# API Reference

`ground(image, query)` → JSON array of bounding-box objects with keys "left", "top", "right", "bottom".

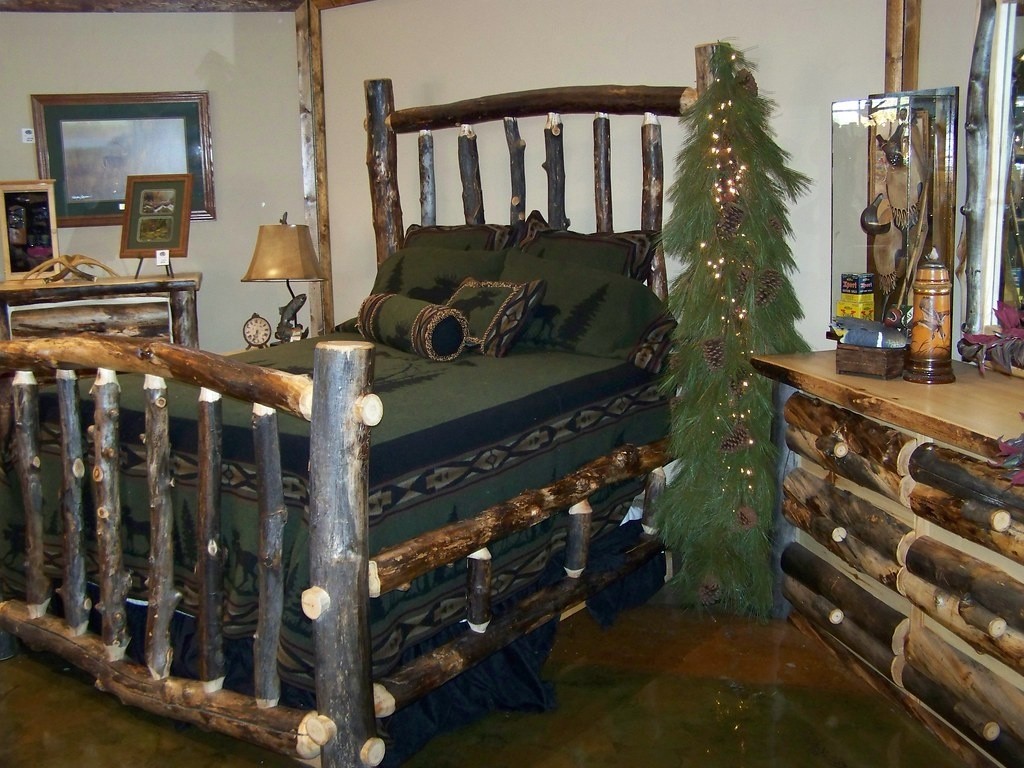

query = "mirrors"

[{"left": 955, "top": 0, "right": 1024, "bottom": 377}]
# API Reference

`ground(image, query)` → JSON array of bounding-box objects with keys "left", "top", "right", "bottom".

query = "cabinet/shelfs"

[
  {"left": 0, "top": 272, "right": 202, "bottom": 383},
  {"left": 750, "top": 350, "right": 1024, "bottom": 768}
]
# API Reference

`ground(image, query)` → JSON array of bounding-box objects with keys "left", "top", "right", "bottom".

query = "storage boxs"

[
  {"left": 837, "top": 273, "right": 874, "bottom": 321},
  {"left": 826, "top": 86, "right": 959, "bottom": 359},
  {"left": 836, "top": 344, "right": 904, "bottom": 380}
]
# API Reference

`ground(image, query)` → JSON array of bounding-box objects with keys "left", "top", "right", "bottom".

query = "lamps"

[{"left": 241, "top": 212, "right": 327, "bottom": 347}]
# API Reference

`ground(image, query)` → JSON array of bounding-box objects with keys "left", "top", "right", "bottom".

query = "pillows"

[{"left": 357, "top": 210, "right": 662, "bottom": 361}]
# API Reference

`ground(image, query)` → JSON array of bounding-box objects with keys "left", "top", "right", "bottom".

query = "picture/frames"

[
  {"left": 120, "top": 174, "right": 192, "bottom": 258},
  {"left": 31, "top": 90, "right": 216, "bottom": 228}
]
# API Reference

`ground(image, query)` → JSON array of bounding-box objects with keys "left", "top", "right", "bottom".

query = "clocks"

[{"left": 243, "top": 313, "right": 271, "bottom": 350}]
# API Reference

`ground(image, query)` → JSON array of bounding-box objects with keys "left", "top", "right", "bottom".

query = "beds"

[{"left": 0, "top": 42, "right": 776, "bottom": 768}]
